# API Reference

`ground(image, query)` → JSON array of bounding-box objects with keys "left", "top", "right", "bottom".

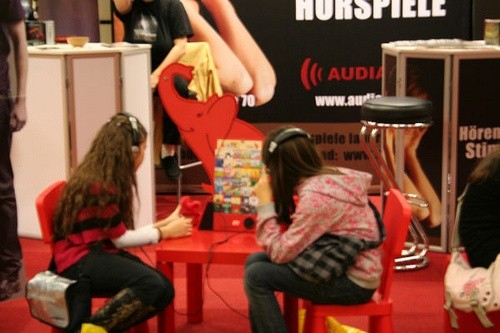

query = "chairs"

[
  {"left": 284, "top": 188, "right": 413, "bottom": 333},
  {"left": 34, "top": 179, "right": 72, "bottom": 275}
]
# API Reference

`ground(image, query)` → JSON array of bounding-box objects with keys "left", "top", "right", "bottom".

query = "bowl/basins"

[{"left": 66, "top": 36, "right": 89, "bottom": 47}]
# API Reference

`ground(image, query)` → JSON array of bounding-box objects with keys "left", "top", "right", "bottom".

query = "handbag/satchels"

[
  {"left": 443, "top": 251, "right": 500, "bottom": 330},
  {"left": 286, "top": 230, "right": 365, "bottom": 287},
  {"left": 25, "top": 270, "right": 81, "bottom": 331}
]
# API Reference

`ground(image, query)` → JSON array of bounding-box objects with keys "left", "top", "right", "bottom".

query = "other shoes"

[
  {"left": 159, "top": 152, "right": 180, "bottom": 178},
  {"left": 0, "top": 279, "right": 22, "bottom": 301}
]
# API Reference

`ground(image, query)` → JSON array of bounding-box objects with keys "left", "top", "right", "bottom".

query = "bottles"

[{"left": 28, "top": 0, "right": 39, "bottom": 20}]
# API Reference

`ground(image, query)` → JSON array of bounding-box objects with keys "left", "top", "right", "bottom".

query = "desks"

[
  {"left": 381, "top": 39, "right": 500, "bottom": 253},
  {"left": 12, "top": 40, "right": 156, "bottom": 240},
  {"left": 155, "top": 196, "right": 299, "bottom": 333}
]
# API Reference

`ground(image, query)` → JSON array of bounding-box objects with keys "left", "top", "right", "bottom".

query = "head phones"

[
  {"left": 265, "top": 128, "right": 311, "bottom": 173},
  {"left": 120, "top": 112, "right": 141, "bottom": 157}
]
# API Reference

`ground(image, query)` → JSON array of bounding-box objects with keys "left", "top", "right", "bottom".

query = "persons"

[
  {"left": 112, "top": 0, "right": 277, "bottom": 106},
  {"left": 0, "top": 0, "right": 28, "bottom": 301},
  {"left": 459, "top": 146, "right": 500, "bottom": 333},
  {"left": 124, "top": 0, "right": 188, "bottom": 181},
  {"left": 51, "top": 112, "right": 194, "bottom": 333},
  {"left": 242, "top": 123, "right": 383, "bottom": 333}
]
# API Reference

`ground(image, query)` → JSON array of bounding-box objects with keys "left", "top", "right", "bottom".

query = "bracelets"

[{"left": 157, "top": 227, "right": 163, "bottom": 243}]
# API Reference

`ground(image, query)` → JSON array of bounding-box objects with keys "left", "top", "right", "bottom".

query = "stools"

[{"left": 357, "top": 95, "right": 443, "bottom": 271}]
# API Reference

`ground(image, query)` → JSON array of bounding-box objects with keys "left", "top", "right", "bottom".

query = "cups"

[{"left": 484, "top": 19, "right": 500, "bottom": 45}]
